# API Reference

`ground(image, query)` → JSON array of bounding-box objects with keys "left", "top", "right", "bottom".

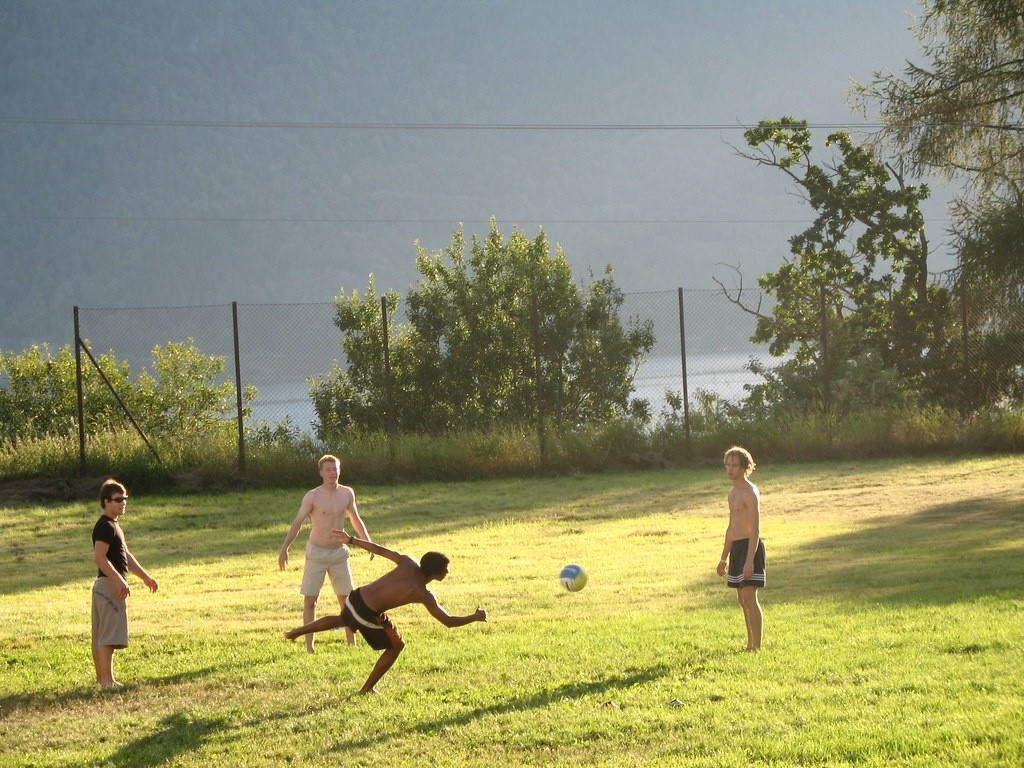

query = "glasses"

[{"left": 107, "top": 495, "right": 128, "bottom": 502}]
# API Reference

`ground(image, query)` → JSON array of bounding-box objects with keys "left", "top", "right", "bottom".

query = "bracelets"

[
  {"left": 720, "top": 560, "right": 728, "bottom": 563},
  {"left": 349, "top": 536, "right": 355, "bottom": 545}
]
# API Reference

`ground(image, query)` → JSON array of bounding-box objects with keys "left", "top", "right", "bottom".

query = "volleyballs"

[{"left": 559, "top": 564, "right": 588, "bottom": 592}]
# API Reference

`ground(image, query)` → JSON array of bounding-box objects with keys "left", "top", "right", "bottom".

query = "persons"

[
  {"left": 277, "top": 454, "right": 374, "bottom": 656},
  {"left": 282, "top": 529, "right": 489, "bottom": 697},
  {"left": 91, "top": 480, "right": 158, "bottom": 687},
  {"left": 717, "top": 448, "right": 766, "bottom": 650}
]
